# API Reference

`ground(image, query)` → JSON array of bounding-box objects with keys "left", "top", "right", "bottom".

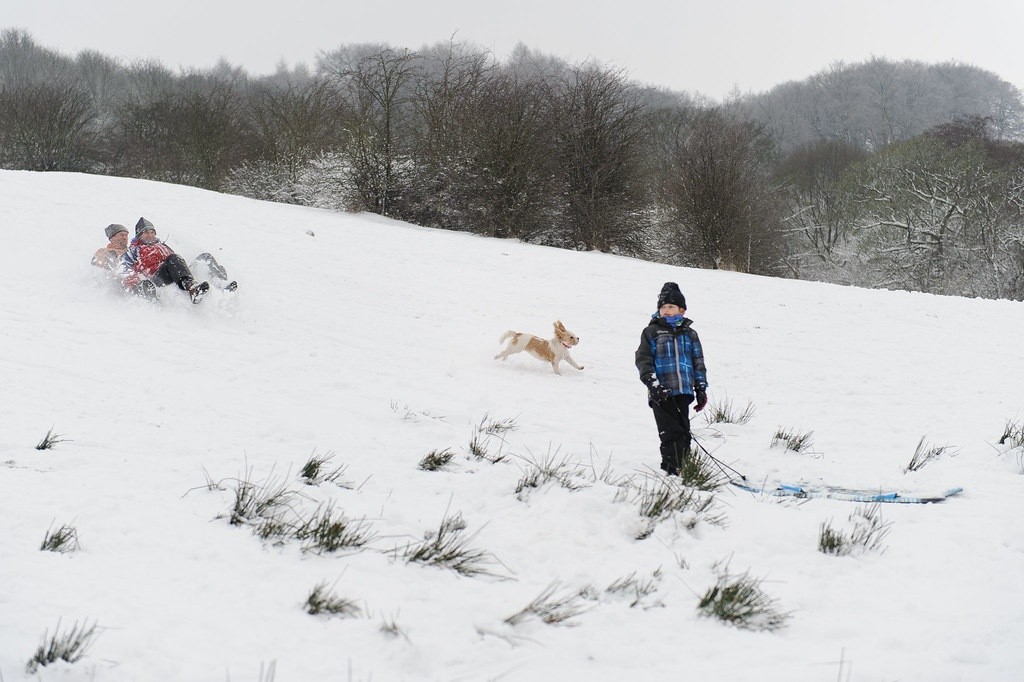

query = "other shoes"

[
  {"left": 142, "top": 280, "right": 162, "bottom": 303},
  {"left": 190, "top": 281, "right": 209, "bottom": 304},
  {"left": 225, "top": 281, "right": 237, "bottom": 292}
]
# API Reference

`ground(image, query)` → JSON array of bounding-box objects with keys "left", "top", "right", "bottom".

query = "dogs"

[{"left": 493, "top": 320, "right": 584, "bottom": 376}]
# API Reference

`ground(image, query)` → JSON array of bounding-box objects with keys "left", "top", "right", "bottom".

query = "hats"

[
  {"left": 136, "top": 217, "right": 156, "bottom": 237},
  {"left": 658, "top": 282, "right": 687, "bottom": 310},
  {"left": 105, "top": 224, "right": 129, "bottom": 240}
]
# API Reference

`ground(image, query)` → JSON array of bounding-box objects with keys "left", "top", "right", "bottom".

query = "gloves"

[
  {"left": 692, "top": 391, "right": 707, "bottom": 412},
  {"left": 650, "top": 385, "right": 672, "bottom": 406}
]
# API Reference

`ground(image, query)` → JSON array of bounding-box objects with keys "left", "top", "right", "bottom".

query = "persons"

[
  {"left": 91, "top": 217, "right": 238, "bottom": 307},
  {"left": 634, "top": 281, "right": 709, "bottom": 480}
]
